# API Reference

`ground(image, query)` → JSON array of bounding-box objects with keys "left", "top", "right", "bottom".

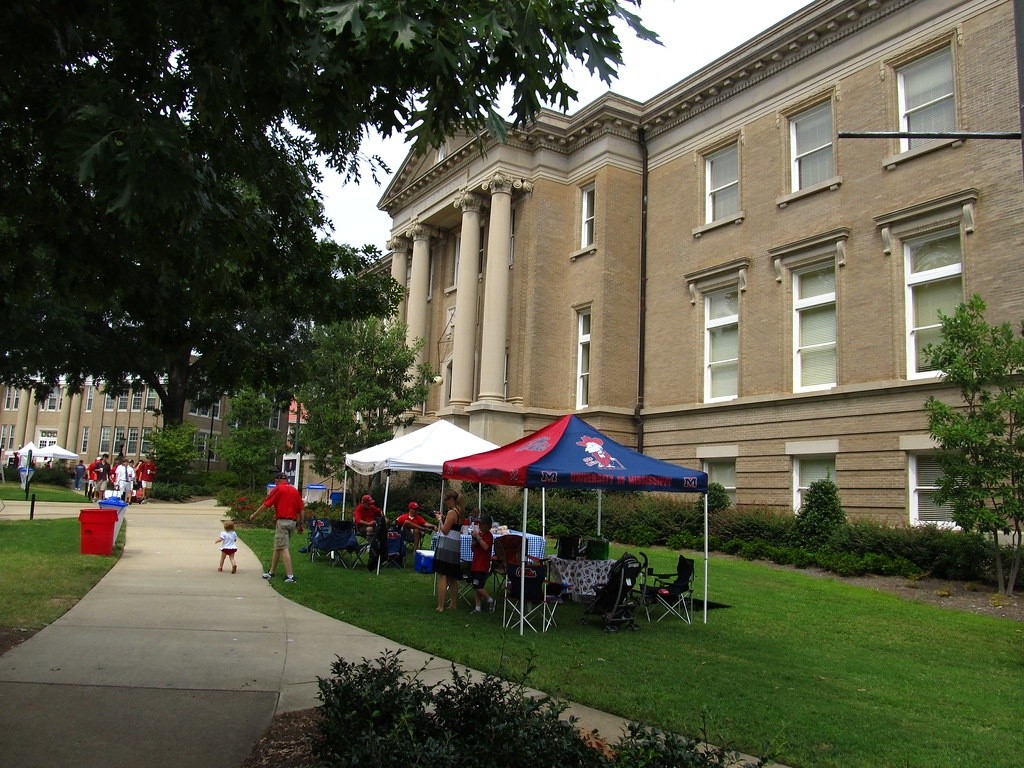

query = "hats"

[
  {"left": 408, "top": 502, "right": 419, "bottom": 509},
  {"left": 475, "top": 514, "right": 492, "bottom": 524},
  {"left": 361, "top": 495, "right": 375, "bottom": 504},
  {"left": 97, "top": 457, "right": 101, "bottom": 460},
  {"left": 275, "top": 473, "right": 288, "bottom": 479}
]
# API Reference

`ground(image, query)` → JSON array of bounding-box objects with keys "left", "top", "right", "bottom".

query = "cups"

[
  {"left": 112, "top": 475, "right": 114, "bottom": 478},
  {"left": 100, "top": 468, "right": 103, "bottom": 472},
  {"left": 435, "top": 511, "right": 440, "bottom": 519},
  {"left": 147, "top": 470, "right": 150, "bottom": 474}
]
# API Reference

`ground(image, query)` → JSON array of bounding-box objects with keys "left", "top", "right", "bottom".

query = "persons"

[
  {"left": 470, "top": 515, "right": 497, "bottom": 615},
  {"left": 353, "top": 495, "right": 389, "bottom": 554},
  {"left": 75, "top": 460, "right": 87, "bottom": 491},
  {"left": 249, "top": 472, "right": 305, "bottom": 584},
  {"left": 32, "top": 460, "right": 50, "bottom": 469},
  {"left": 392, "top": 501, "right": 437, "bottom": 555},
  {"left": 85, "top": 454, "right": 157, "bottom": 505},
  {"left": 216, "top": 521, "right": 237, "bottom": 574},
  {"left": 434, "top": 491, "right": 465, "bottom": 612},
  {"left": 12, "top": 452, "right": 19, "bottom": 467}
]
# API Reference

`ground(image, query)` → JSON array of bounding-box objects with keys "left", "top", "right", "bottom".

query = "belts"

[{"left": 276, "top": 518, "right": 296, "bottom": 521}]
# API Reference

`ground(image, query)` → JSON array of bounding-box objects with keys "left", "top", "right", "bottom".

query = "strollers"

[{"left": 576, "top": 552, "right": 649, "bottom": 633}]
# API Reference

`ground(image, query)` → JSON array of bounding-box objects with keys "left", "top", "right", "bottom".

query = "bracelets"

[
  {"left": 478, "top": 537, "right": 482, "bottom": 542},
  {"left": 438, "top": 518, "right": 441, "bottom": 521}
]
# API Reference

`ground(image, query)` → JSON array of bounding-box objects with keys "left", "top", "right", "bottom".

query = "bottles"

[{"left": 474, "top": 521, "right": 479, "bottom": 534}]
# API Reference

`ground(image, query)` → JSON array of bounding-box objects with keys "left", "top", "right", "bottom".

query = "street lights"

[{"left": 118, "top": 433, "right": 126, "bottom": 455}]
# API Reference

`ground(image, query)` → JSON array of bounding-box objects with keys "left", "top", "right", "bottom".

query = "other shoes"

[
  {"left": 434, "top": 607, "right": 444, "bottom": 614},
  {"left": 425, "top": 528, "right": 432, "bottom": 535},
  {"left": 219, "top": 568, "right": 222, "bottom": 571},
  {"left": 448, "top": 604, "right": 457, "bottom": 611},
  {"left": 367, "top": 546, "right": 370, "bottom": 554},
  {"left": 232, "top": 566, "right": 236, "bottom": 574},
  {"left": 413, "top": 550, "right": 416, "bottom": 556}
]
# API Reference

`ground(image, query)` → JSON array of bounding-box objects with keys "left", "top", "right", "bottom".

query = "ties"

[{"left": 125, "top": 466, "right": 128, "bottom": 482}]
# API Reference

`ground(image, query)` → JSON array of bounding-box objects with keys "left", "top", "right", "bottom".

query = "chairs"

[{"left": 307, "top": 516, "right": 694, "bottom": 632}]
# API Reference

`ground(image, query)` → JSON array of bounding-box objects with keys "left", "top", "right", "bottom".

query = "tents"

[
  {"left": 32, "top": 444, "right": 79, "bottom": 467},
  {"left": 434, "top": 414, "right": 708, "bottom": 623},
  {"left": 18, "top": 440, "right": 38, "bottom": 456},
  {"left": 341, "top": 418, "right": 502, "bottom": 534}
]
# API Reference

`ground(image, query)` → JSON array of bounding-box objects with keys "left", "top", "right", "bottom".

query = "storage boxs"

[{"left": 414, "top": 549, "right": 436, "bottom": 573}]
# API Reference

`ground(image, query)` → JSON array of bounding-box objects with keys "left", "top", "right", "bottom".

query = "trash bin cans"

[
  {"left": 330, "top": 491, "right": 343, "bottom": 507},
  {"left": 79, "top": 509, "right": 118, "bottom": 555}
]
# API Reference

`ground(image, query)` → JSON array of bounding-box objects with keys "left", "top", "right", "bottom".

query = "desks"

[
  {"left": 547, "top": 556, "right": 617, "bottom": 603},
  {"left": 431, "top": 530, "right": 545, "bottom": 562}
]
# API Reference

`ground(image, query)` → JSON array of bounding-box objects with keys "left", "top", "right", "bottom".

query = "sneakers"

[
  {"left": 487, "top": 598, "right": 496, "bottom": 614},
  {"left": 283, "top": 575, "right": 296, "bottom": 584},
  {"left": 262, "top": 570, "right": 274, "bottom": 580},
  {"left": 471, "top": 610, "right": 482, "bottom": 615}
]
